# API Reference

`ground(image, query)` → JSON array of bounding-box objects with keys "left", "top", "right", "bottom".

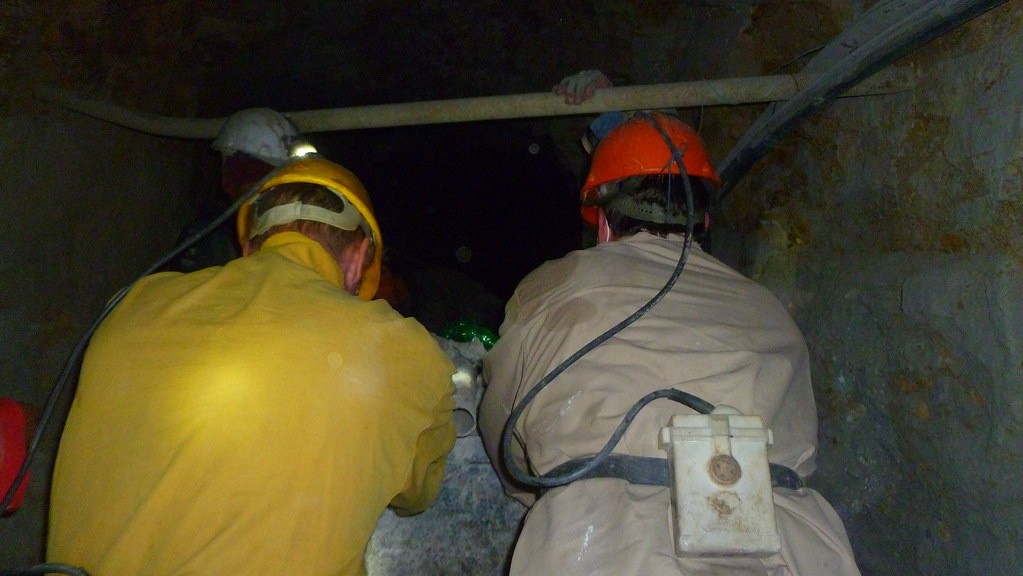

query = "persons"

[{"left": 44, "top": 70, "right": 860, "bottom": 576}]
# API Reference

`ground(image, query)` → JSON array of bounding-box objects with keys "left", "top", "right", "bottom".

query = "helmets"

[
  {"left": 580, "top": 113, "right": 720, "bottom": 223},
  {"left": 210, "top": 108, "right": 298, "bottom": 167},
  {"left": 237, "top": 155, "right": 382, "bottom": 300}
]
